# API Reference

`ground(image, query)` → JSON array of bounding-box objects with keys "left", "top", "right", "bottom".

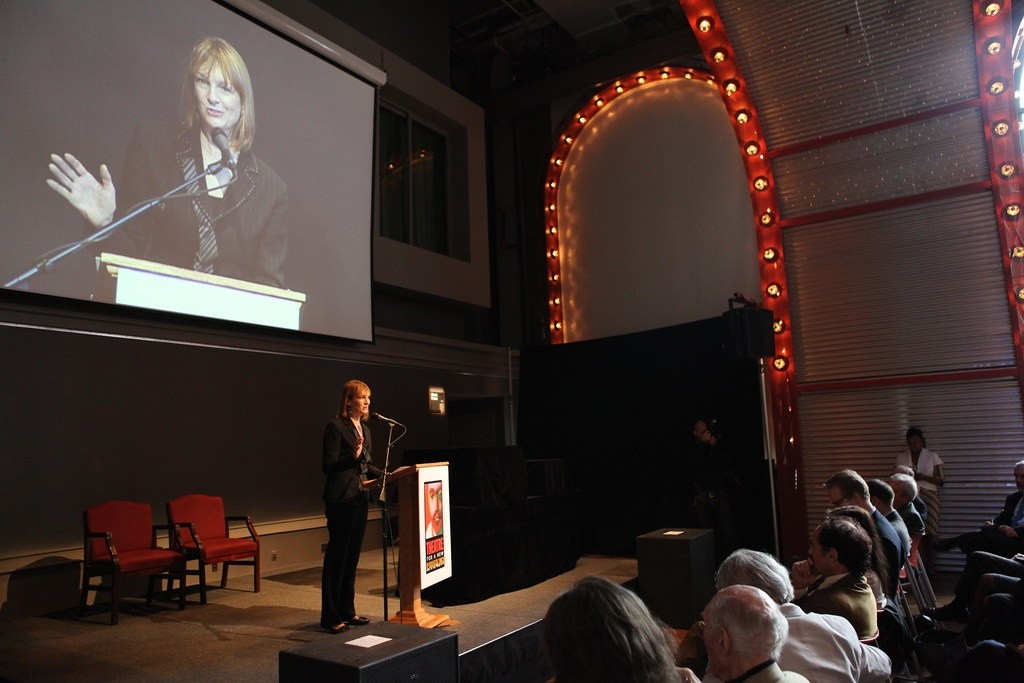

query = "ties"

[
  {"left": 1011, "top": 499, "right": 1024, "bottom": 528},
  {"left": 807, "top": 576, "right": 825, "bottom": 594}
]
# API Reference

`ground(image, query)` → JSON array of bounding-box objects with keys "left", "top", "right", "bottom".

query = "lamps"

[
  {"left": 549, "top": 204, "right": 556, "bottom": 214},
  {"left": 660, "top": 71, "right": 671, "bottom": 80},
  {"left": 997, "top": 161, "right": 1018, "bottom": 180},
  {"left": 684, "top": 71, "right": 694, "bottom": 80},
  {"left": 696, "top": 14, "right": 790, "bottom": 374},
  {"left": 550, "top": 180, "right": 557, "bottom": 190},
  {"left": 636, "top": 76, "right": 647, "bottom": 84},
  {"left": 552, "top": 272, "right": 560, "bottom": 282},
  {"left": 986, "top": 75, "right": 1010, "bottom": 97},
  {"left": 595, "top": 98, "right": 604, "bottom": 107},
  {"left": 982, "top": 36, "right": 1006, "bottom": 56},
  {"left": 979, "top": 0, "right": 1005, "bottom": 17},
  {"left": 991, "top": 119, "right": 1012, "bottom": 138},
  {"left": 554, "top": 321, "right": 562, "bottom": 331},
  {"left": 555, "top": 157, "right": 563, "bottom": 167},
  {"left": 564, "top": 135, "right": 573, "bottom": 144},
  {"left": 578, "top": 115, "right": 587, "bottom": 124},
  {"left": 1000, "top": 202, "right": 1024, "bottom": 222},
  {"left": 551, "top": 248, "right": 559, "bottom": 260},
  {"left": 550, "top": 226, "right": 557, "bottom": 236},
  {"left": 1015, "top": 285, "right": 1024, "bottom": 304},
  {"left": 553, "top": 295, "right": 560, "bottom": 307},
  {"left": 1010, "top": 243, "right": 1024, "bottom": 263},
  {"left": 615, "top": 85, "right": 625, "bottom": 94}
]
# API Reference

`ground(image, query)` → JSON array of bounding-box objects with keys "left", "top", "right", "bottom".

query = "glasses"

[
  {"left": 833, "top": 496, "right": 847, "bottom": 508},
  {"left": 699, "top": 621, "right": 718, "bottom": 632}
]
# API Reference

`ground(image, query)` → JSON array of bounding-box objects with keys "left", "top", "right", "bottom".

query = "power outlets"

[
  {"left": 271, "top": 550, "right": 278, "bottom": 562},
  {"left": 321, "top": 542, "right": 329, "bottom": 553},
  {"left": 211, "top": 563, "right": 218, "bottom": 572}
]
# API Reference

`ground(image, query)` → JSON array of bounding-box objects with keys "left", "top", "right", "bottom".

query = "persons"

[
  {"left": 320, "top": 379, "right": 411, "bottom": 634},
  {"left": 47, "top": 36, "right": 290, "bottom": 284},
  {"left": 687, "top": 420, "right": 740, "bottom": 552},
  {"left": 541, "top": 427, "right": 1024, "bottom": 683}
]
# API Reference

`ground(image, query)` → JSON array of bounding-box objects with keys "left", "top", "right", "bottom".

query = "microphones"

[
  {"left": 212, "top": 128, "right": 236, "bottom": 173},
  {"left": 372, "top": 413, "right": 397, "bottom": 426}
]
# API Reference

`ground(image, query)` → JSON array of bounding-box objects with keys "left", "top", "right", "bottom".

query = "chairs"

[
  {"left": 165, "top": 494, "right": 261, "bottom": 605},
  {"left": 78, "top": 499, "right": 187, "bottom": 627},
  {"left": 861, "top": 531, "right": 946, "bottom": 683}
]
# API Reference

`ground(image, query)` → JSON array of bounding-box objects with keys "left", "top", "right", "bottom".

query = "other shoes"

[
  {"left": 933, "top": 536, "right": 961, "bottom": 551},
  {"left": 321, "top": 623, "right": 350, "bottom": 634},
  {"left": 342, "top": 617, "right": 370, "bottom": 624},
  {"left": 922, "top": 604, "right": 970, "bottom": 624}
]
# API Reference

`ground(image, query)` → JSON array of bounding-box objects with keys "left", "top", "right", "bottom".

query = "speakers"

[
  {"left": 279, "top": 620, "right": 460, "bottom": 683},
  {"left": 638, "top": 526, "right": 717, "bottom": 629}
]
